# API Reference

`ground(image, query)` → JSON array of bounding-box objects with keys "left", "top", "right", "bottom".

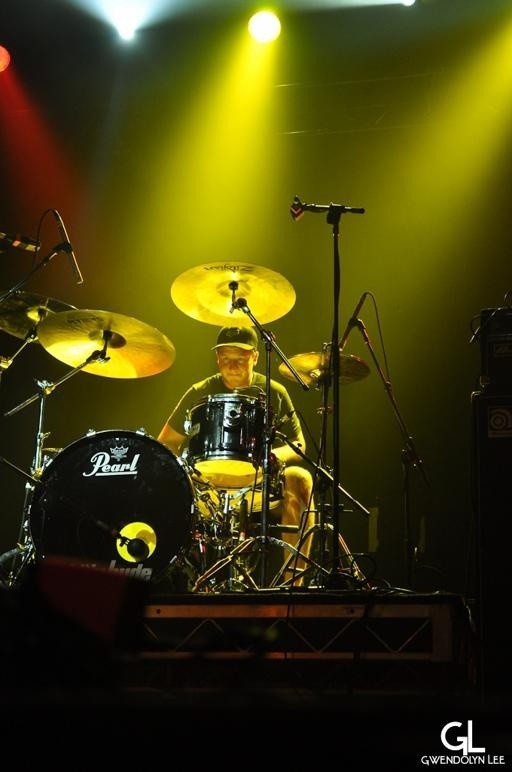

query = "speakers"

[{"left": 466, "top": 392, "right": 512, "bottom": 772}]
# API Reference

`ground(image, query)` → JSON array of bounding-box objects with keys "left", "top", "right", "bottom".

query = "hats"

[{"left": 211, "top": 326, "right": 258, "bottom": 351}]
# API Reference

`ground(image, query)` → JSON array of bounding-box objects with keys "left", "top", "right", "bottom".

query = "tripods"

[
  {"left": 190, "top": 309, "right": 328, "bottom": 591},
  {"left": 272, "top": 226, "right": 378, "bottom": 590}
]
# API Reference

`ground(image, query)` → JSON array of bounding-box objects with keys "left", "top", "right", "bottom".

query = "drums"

[
  {"left": 22, "top": 427, "right": 205, "bottom": 596},
  {"left": 187, "top": 393, "right": 268, "bottom": 490},
  {"left": 218, "top": 459, "right": 286, "bottom": 512},
  {"left": 30, "top": 445, "right": 62, "bottom": 493}
]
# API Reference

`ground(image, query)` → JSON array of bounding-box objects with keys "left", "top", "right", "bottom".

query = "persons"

[{"left": 158, "top": 323, "right": 315, "bottom": 588}]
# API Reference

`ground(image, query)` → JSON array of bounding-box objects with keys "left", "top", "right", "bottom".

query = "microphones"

[
  {"left": 236, "top": 296, "right": 248, "bottom": 313},
  {"left": 312, "top": 201, "right": 368, "bottom": 218},
  {"left": 56, "top": 210, "right": 84, "bottom": 283},
  {"left": 339, "top": 295, "right": 365, "bottom": 352}
]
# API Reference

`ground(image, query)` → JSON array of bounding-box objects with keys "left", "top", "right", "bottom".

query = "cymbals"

[
  {"left": 0, "top": 287, "right": 78, "bottom": 344},
  {"left": 170, "top": 261, "right": 296, "bottom": 327},
  {"left": 36, "top": 309, "right": 176, "bottom": 380},
  {"left": 278, "top": 351, "right": 369, "bottom": 387}
]
